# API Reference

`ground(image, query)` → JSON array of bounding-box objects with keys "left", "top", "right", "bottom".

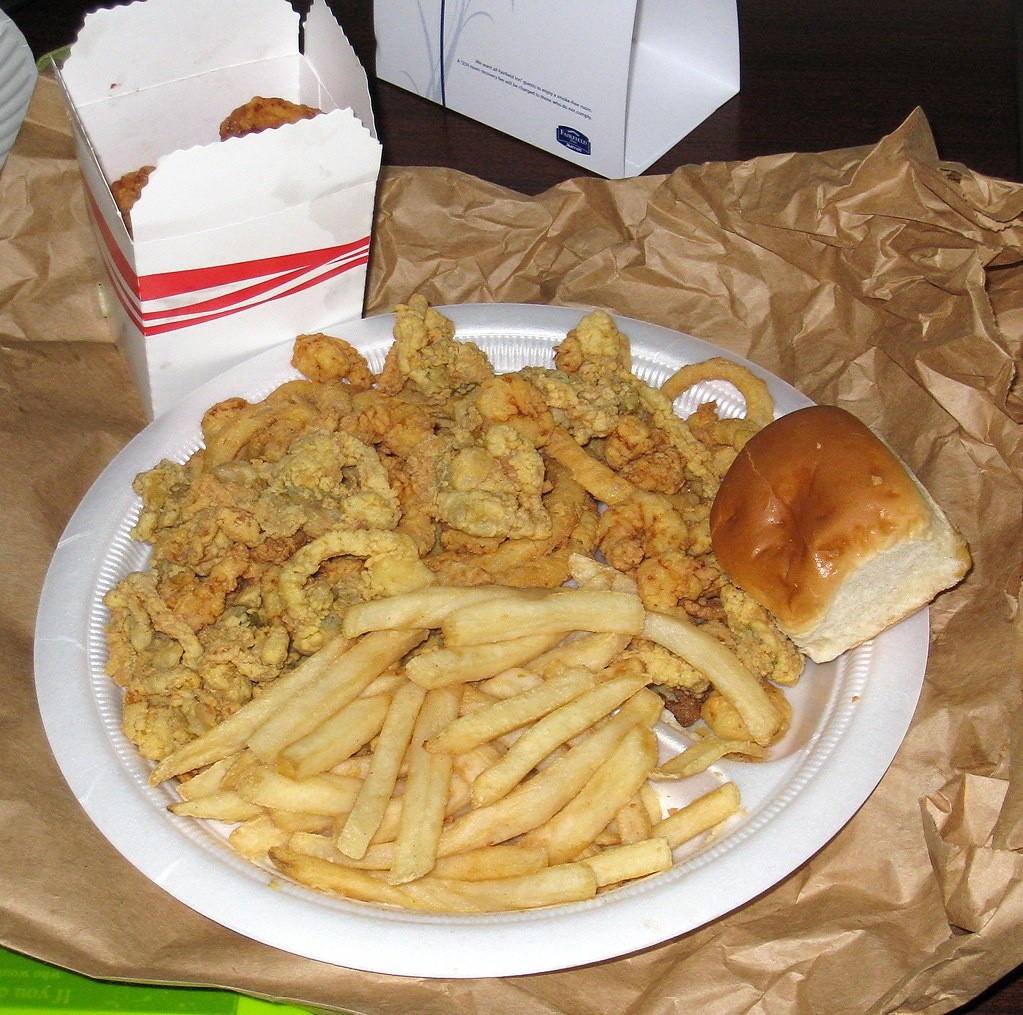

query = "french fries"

[{"left": 147, "top": 553, "right": 787, "bottom": 913}]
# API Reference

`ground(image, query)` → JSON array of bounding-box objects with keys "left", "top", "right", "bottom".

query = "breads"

[{"left": 712, "top": 403, "right": 973, "bottom": 664}]
